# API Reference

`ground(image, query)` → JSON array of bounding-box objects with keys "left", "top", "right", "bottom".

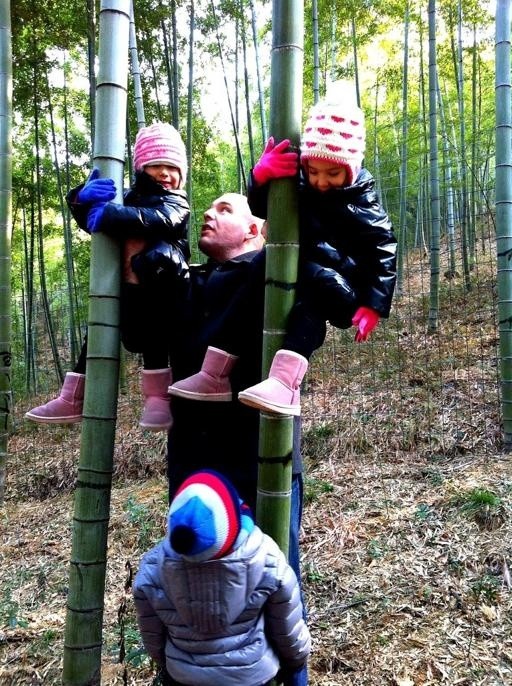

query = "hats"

[
  {"left": 299, "top": 80, "right": 365, "bottom": 186},
  {"left": 167, "top": 470, "right": 255, "bottom": 563},
  {"left": 134, "top": 122, "right": 188, "bottom": 188}
]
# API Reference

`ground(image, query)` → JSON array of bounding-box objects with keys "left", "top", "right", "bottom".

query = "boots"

[
  {"left": 168, "top": 346, "right": 238, "bottom": 402},
  {"left": 140, "top": 368, "right": 174, "bottom": 430},
  {"left": 238, "top": 349, "right": 309, "bottom": 416},
  {"left": 26, "top": 372, "right": 85, "bottom": 423}
]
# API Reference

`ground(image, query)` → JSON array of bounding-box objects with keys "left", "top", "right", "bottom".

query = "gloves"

[
  {"left": 253, "top": 135, "right": 298, "bottom": 185},
  {"left": 78, "top": 169, "right": 116, "bottom": 231},
  {"left": 352, "top": 307, "right": 379, "bottom": 342}
]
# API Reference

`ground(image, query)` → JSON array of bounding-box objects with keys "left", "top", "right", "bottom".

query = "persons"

[
  {"left": 166, "top": 96, "right": 398, "bottom": 418},
  {"left": 168, "top": 193, "right": 305, "bottom": 686},
  {"left": 131, "top": 470, "right": 312, "bottom": 686},
  {"left": 26, "top": 120, "right": 190, "bottom": 432}
]
